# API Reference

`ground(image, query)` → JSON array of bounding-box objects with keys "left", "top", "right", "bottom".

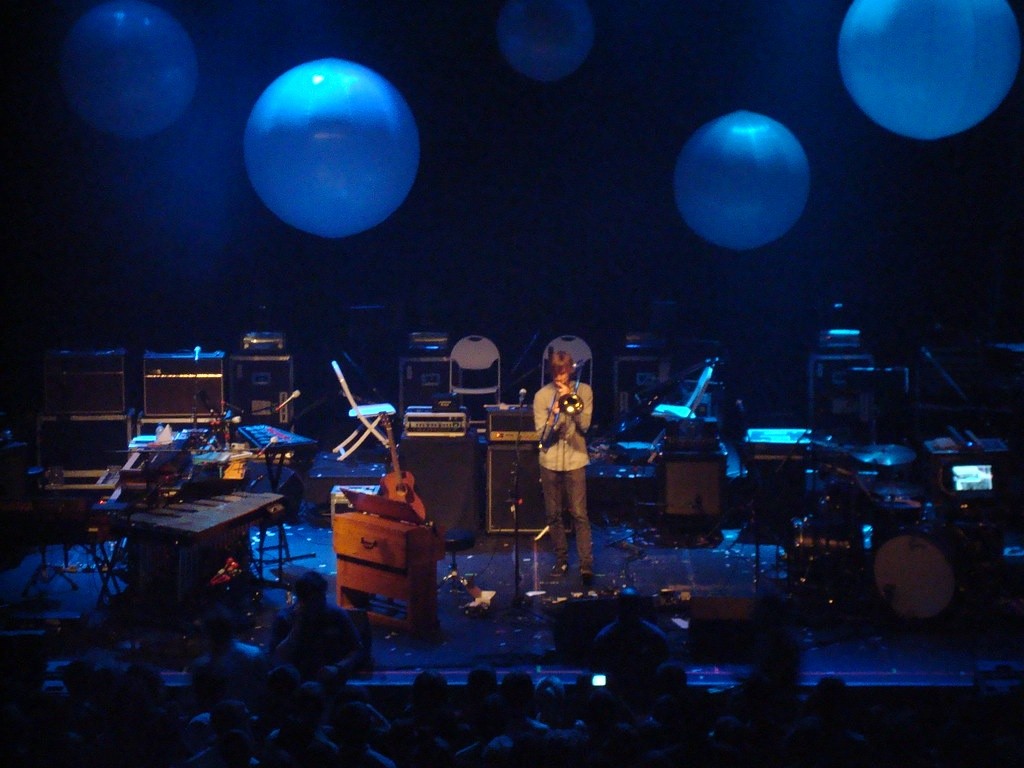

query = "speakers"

[
  {"left": 144, "top": 350, "right": 227, "bottom": 416},
  {"left": 653, "top": 451, "right": 724, "bottom": 518},
  {"left": 42, "top": 349, "right": 131, "bottom": 414},
  {"left": 555, "top": 595, "right": 656, "bottom": 656},
  {"left": 686, "top": 595, "right": 759, "bottom": 651},
  {"left": 487, "top": 444, "right": 574, "bottom": 534}
]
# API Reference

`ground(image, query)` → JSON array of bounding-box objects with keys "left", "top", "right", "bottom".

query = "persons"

[
  {"left": 532, "top": 350, "right": 594, "bottom": 586},
  {"left": 0, "top": 570, "right": 1024, "bottom": 768}
]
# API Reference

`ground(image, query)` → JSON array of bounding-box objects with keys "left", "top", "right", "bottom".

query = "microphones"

[
  {"left": 194, "top": 347, "right": 202, "bottom": 361},
  {"left": 210, "top": 416, "right": 241, "bottom": 425},
  {"left": 519, "top": 388, "right": 527, "bottom": 402},
  {"left": 275, "top": 390, "right": 301, "bottom": 411}
]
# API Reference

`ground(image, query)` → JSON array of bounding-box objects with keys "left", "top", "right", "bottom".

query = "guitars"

[{"left": 378, "top": 412, "right": 427, "bottom": 519}]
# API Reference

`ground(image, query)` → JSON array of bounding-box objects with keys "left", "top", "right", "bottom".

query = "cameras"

[{"left": 590, "top": 675, "right": 607, "bottom": 686}]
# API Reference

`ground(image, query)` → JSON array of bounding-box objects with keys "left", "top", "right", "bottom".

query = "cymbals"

[
  {"left": 872, "top": 481, "right": 923, "bottom": 497},
  {"left": 849, "top": 441, "right": 916, "bottom": 467},
  {"left": 878, "top": 498, "right": 922, "bottom": 509}
]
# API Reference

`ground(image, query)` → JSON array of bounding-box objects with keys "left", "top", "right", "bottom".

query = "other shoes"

[
  {"left": 580, "top": 566, "right": 592, "bottom": 578},
  {"left": 551, "top": 559, "right": 568, "bottom": 577}
]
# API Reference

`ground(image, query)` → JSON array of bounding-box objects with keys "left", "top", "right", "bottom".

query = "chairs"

[
  {"left": 330, "top": 360, "right": 396, "bottom": 461},
  {"left": 651, "top": 364, "right": 714, "bottom": 449},
  {"left": 450, "top": 334, "right": 502, "bottom": 407},
  {"left": 540, "top": 336, "right": 594, "bottom": 387}
]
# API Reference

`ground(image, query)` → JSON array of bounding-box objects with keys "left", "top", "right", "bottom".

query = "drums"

[{"left": 872, "top": 520, "right": 971, "bottom": 622}]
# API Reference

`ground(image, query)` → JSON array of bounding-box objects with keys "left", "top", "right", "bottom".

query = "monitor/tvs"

[{"left": 951, "top": 464, "right": 994, "bottom": 492}]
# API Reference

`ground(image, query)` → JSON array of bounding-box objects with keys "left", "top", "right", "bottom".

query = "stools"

[{"left": 91, "top": 503, "right": 131, "bottom": 611}]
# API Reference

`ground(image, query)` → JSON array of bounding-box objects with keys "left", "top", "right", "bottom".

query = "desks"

[
  {"left": 33, "top": 408, "right": 134, "bottom": 463},
  {"left": 136, "top": 409, "right": 234, "bottom": 446}
]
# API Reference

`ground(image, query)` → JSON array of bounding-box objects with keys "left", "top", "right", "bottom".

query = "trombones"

[{"left": 537, "top": 357, "right": 584, "bottom": 446}]
